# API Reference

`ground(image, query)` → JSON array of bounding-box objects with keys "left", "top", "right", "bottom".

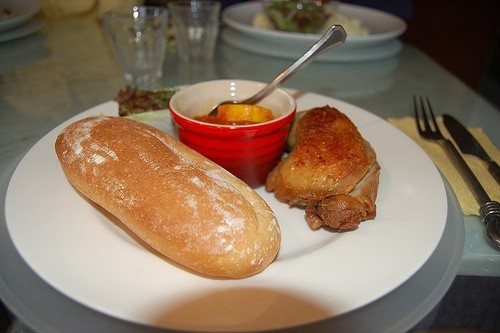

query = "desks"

[{"left": 0, "top": 13, "right": 500, "bottom": 275}]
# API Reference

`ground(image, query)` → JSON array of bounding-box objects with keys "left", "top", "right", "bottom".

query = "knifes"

[{"left": 440, "top": 112, "right": 500, "bottom": 185}]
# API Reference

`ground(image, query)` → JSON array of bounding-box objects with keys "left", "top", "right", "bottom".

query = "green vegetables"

[
  {"left": 264, "top": 0, "right": 326, "bottom": 33},
  {"left": 126, "top": 85, "right": 181, "bottom": 114}
]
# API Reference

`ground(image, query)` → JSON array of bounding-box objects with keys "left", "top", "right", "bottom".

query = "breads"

[{"left": 55, "top": 115, "right": 281, "bottom": 278}]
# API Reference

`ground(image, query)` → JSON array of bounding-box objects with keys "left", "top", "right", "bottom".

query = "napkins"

[{"left": 389, "top": 116, "right": 500, "bottom": 216}]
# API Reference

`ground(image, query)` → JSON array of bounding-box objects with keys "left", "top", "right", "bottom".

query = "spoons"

[{"left": 207, "top": 24, "right": 347, "bottom": 118}]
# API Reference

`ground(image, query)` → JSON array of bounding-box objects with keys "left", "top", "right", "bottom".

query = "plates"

[
  {"left": 222, "top": 0, "right": 406, "bottom": 50},
  {"left": 0, "top": 6, "right": 40, "bottom": 30},
  {"left": 5, "top": 83, "right": 447, "bottom": 333}
]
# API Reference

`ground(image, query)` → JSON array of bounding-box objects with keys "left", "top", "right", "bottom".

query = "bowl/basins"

[{"left": 168, "top": 78, "right": 296, "bottom": 191}]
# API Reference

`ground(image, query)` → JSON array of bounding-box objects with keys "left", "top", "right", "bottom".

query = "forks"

[{"left": 411, "top": 94, "right": 500, "bottom": 246}]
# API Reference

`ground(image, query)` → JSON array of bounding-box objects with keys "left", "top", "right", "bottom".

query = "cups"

[
  {"left": 102, "top": 6, "right": 168, "bottom": 84},
  {"left": 168, "top": 0, "right": 221, "bottom": 62}
]
